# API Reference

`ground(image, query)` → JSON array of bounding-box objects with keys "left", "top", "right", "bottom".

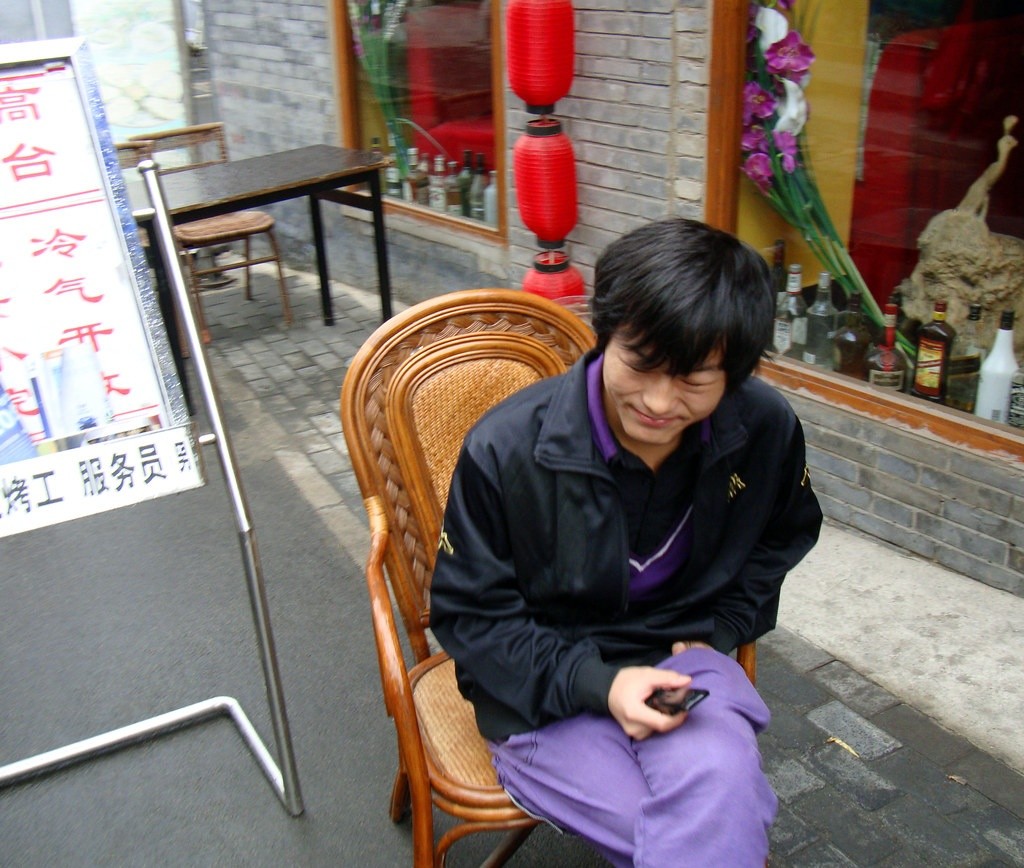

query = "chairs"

[
  {"left": 340, "top": 288, "right": 756, "bottom": 868},
  {"left": 131, "top": 114, "right": 292, "bottom": 344},
  {"left": 115, "top": 140, "right": 156, "bottom": 289}
]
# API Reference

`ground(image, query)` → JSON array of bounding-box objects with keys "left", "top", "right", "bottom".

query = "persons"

[{"left": 427, "top": 217, "right": 823, "bottom": 868}]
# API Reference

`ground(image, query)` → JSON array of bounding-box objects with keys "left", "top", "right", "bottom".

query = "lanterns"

[
  {"left": 505, "top": 0, "right": 575, "bottom": 114},
  {"left": 514, "top": 119, "right": 578, "bottom": 248},
  {"left": 522, "top": 250, "right": 586, "bottom": 318}
]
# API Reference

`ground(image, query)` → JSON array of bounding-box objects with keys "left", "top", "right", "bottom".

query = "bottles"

[
  {"left": 484, "top": 170, "right": 498, "bottom": 226},
  {"left": 416, "top": 152, "right": 431, "bottom": 206},
  {"left": 370, "top": 137, "right": 387, "bottom": 194},
  {"left": 766, "top": 238, "right": 787, "bottom": 299},
  {"left": 866, "top": 303, "right": 907, "bottom": 393},
  {"left": 429, "top": 156, "right": 447, "bottom": 213},
  {"left": 445, "top": 161, "right": 463, "bottom": 216},
  {"left": 836, "top": 291, "right": 868, "bottom": 334},
  {"left": 889, "top": 291, "right": 907, "bottom": 329},
  {"left": 802, "top": 272, "right": 837, "bottom": 371},
  {"left": 469, "top": 153, "right": 489, "bottom": 220},
  {"left": 833, "top": 302, "right": 868, "bottom": 381},
  {"left": 386, "top": 133, "right": 402, "bottom": 195},
  {"left": 403, "top": 148, "right": 419, "bottom": 203},
  {"left": 459, "top": 151, "right": 473, "bottom": 217},
  {"left": 944, "top": 304, "right": 981, "bottom": 414},
  {"left": 910, "top": 300, "right": 957, "bottom": 405},
  {"left": 776, "top": 265, "right": 808, "bottom": 360},
  {"left": 973, "top": 310, "right": 1019, "bottom": 423}
]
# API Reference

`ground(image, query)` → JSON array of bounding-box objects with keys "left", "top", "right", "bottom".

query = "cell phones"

[{"left": 645, "top": 688, "right": 709, "bottom": 715}]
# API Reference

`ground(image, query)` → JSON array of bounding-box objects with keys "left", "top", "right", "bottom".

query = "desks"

[{"left": 118, "top": 143, "right": 393, "bottom": 416}]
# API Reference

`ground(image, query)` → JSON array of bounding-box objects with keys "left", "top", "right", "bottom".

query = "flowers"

[
  {"left": 741, "top": 0, "right": 915, "bottom": 353},
  {"left": 348, "top": 0, "right": 410, "bottom": 179}
]
